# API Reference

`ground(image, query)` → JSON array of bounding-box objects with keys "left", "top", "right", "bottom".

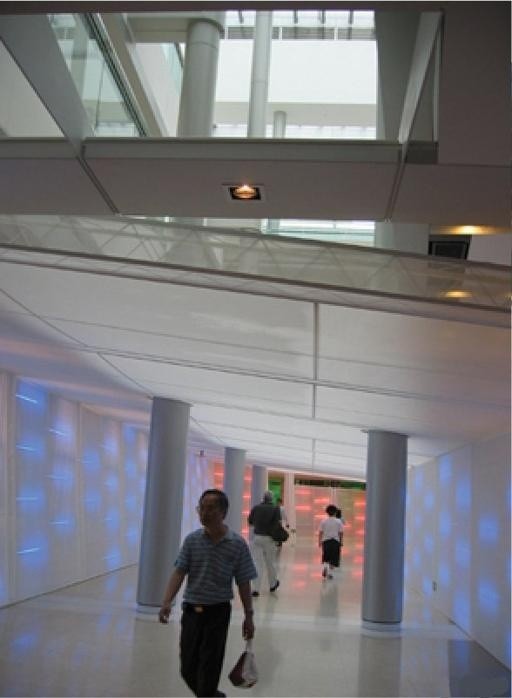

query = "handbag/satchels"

[{"left": 271, "top": 519, "right": 289, "bottom": 542}]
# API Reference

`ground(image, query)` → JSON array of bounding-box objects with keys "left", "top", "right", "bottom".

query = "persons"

[
  {"left": 254, "top": 596, "right": 286, "bottom": 697},
  {"left": 317, "top": 504, "right": 344, "bottom": 580},
  {"left": 313, "top": 580, "right": 341, "bottom": 653},
  {"left": 158, "top": 488, "right": 258, "bottom": 697},
  {"left": 335, "top": 508, "right": 344, "bottom": 525},
  {"left": 272, "top": 497, "right": 290, "bottom": 547},
  {"left": 246, "top": 490, "right": 282, "bottom": 596}
]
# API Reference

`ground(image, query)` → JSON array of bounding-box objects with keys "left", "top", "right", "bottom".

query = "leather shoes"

[
  {"left": 270, "top": 580, "right": 279, "bottom": 591},
  {"left": 252, "top": 591, "right": 258, "bottom": 596}
]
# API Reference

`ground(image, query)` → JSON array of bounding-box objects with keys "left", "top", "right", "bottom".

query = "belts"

[{"left": 185, "top": 603, "right": 214, "bottom": 613}]
